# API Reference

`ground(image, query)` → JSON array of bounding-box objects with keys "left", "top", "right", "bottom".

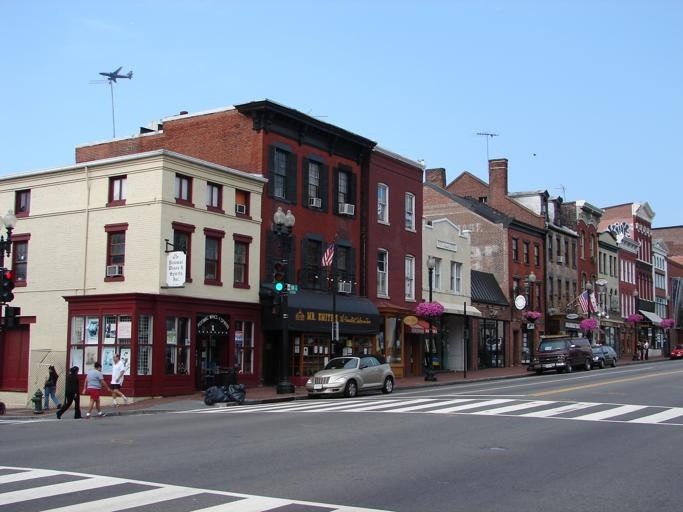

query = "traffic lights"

[
  {"left": 0, "top": 267, "right": 15, "bottom": 302},
  {"left": 7, "top": 307, "right": 21, "bottom": 328},
  {"left": 271, "top": 262, "right": 287, "bottom": 294}
]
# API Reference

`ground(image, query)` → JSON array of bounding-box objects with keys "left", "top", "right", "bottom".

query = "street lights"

[
  {"left": 585, "top": 280, "right": 592, "bottom": 337},
  {"left": 426, "top": 257, "right": 436, "bottom": 381},
  {"left": 271, "top": 207, "right": 295, "bottom": 394},
  {"left": 528, "top": 271, "right": 536, "bottom": 371},
  {"left": 632, "top": 288, "right": 639, "bottom": 361},
  {"left": 1, "top": 209, "right": 17, "bottom": 268}
]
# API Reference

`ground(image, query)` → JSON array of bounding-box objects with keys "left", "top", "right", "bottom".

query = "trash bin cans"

[{"left": 218, "top": 367, "right": 237, "bottom": 391}]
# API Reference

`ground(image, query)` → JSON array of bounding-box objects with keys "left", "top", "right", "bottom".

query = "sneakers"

[
  {"left": 96, "top": 413, "right": 105, "bottom": 417},
  {"left": 86, "top": 413, "right": 91, "bottom": 417}
]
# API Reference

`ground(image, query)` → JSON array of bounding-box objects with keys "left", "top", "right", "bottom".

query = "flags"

[
  {"left": 588, "top": 292, "right": 598, "bottom": 314},
  {"left": 318, "top": 241, "right": 335, "bottom": 267},
  {"left": 576, "top": 291, "right": 591, "bottom": 313}
]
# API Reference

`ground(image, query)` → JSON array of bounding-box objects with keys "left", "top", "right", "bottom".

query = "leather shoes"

[
  {"left": 74, "top": 417, "right": 82, "bottom": 419},
  {"left": 56, "top": 412, "right": 60, "bottom": 419}
]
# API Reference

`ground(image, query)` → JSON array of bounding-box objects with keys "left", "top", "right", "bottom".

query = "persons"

[
  {"left": 642, "top": 339, "right": 649, "bottom": 360},
  {"left": 41, "top": 365, "right": 62, "bottom": 411},
  {"left": 635, "top": 338, "right": 643, "bottom": 360},
  {"left": 108, "top": 353, "right": 129, "bottom": 410},
  {"left": 80, "top": 361, "right": 112, "bottom": 418},
  {"left": 55, "top": 365, "right": 84, "bottom": 419}
]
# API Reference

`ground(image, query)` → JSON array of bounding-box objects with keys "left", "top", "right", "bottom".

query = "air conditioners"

[
  {"left": 308, "top": 198, "right": 321, "bottom": 208},
  {"left": 338, "top": 283, "right": 351, "bottom": 293},
  {"left": 107, "top": 265, "right": 122, "bottom": 276},
  {"left": 235, "top": 204, "right": 246, "bottom": 214},
  {"left": 557, "top": 256, "right": 565, "bottom": 263},
  {"left": 339, "top": 204, "right": 355, "bottom": 216}
]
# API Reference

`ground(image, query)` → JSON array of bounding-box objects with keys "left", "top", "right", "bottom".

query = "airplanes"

[{"left": 99, "top": 66, "right": 134, "bottom": 83}]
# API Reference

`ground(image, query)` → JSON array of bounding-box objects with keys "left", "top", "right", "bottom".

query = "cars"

[
  {"left": 304, "top": 355, "right": 396, "bottom": 398},
  {"left": 486, "top": 337, "right": 502, "bottom": 352},
  {"left": 670, "top": 344, "right": 683, "bottom": 359},
  {"left": 533, "top": 337, "right": 617, "bottom": 375}
]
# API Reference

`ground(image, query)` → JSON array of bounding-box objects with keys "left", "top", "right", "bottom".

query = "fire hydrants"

[{"left": 31, "top": 389, "right": 44, "bottom": 414}]
